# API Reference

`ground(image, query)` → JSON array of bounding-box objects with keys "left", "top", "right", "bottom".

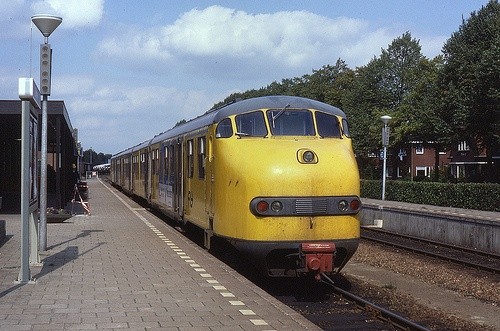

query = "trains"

[{"left": 109, "top": 95, "right": 363, "bottom": 283}]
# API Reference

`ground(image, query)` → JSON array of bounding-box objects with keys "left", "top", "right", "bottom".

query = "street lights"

[
  {"left": 30, "top": 14, "right": 63, "bottom": 251},
  {"left": 380, "top": 115, "right": 392, "bottom": 200}
]
